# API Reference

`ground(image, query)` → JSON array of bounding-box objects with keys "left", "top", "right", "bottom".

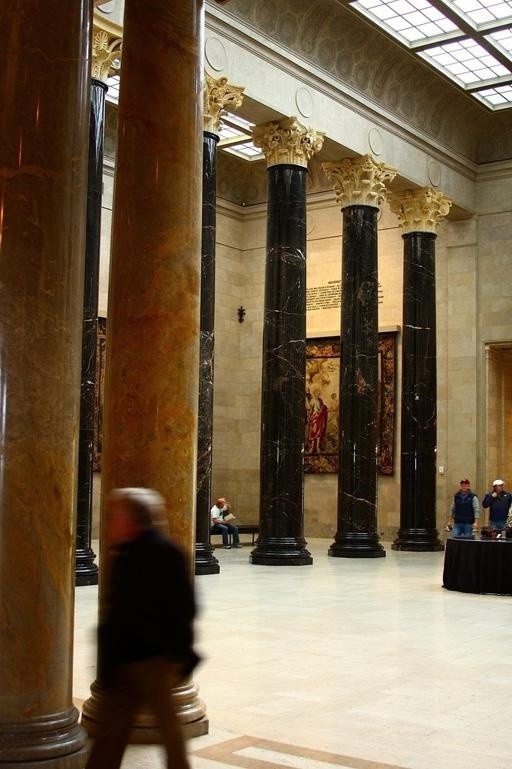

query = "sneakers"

[{"left": 222, "top": 544, "right": 243, "bottom": 549}]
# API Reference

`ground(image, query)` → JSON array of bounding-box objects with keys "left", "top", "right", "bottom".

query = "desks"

[{"left": 446, "top": 538, "right": 512, "bottom": 595}]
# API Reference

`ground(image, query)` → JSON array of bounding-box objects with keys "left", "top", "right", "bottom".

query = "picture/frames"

[{"left": 303, "top": 333, "right": 397, "bottom": 475}]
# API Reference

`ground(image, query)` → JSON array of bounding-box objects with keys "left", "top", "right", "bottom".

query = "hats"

[
  {"left": 492, "top": 480, "right": 505, "bottom": 487},
  {"left": 460, "top": 479, "right": 469, "bottom": 484}
]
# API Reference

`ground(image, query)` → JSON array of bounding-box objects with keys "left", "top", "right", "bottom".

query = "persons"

[
  {"left": 447, "top": 479, "right": 480, "bottom": 537},
  {"left": 210, "top": 498, "right": 243, "bottom": 549},
  {"left": 482, "top": 479, "right": 512, "bottom": 529},
  {"left": 86, "top": 488, "right": 200, "bottom": 768}
]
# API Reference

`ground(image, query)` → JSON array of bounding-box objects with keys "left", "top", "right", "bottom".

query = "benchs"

[{"left": 210, "top": 525, "right": 259, "bottom": 546}]
